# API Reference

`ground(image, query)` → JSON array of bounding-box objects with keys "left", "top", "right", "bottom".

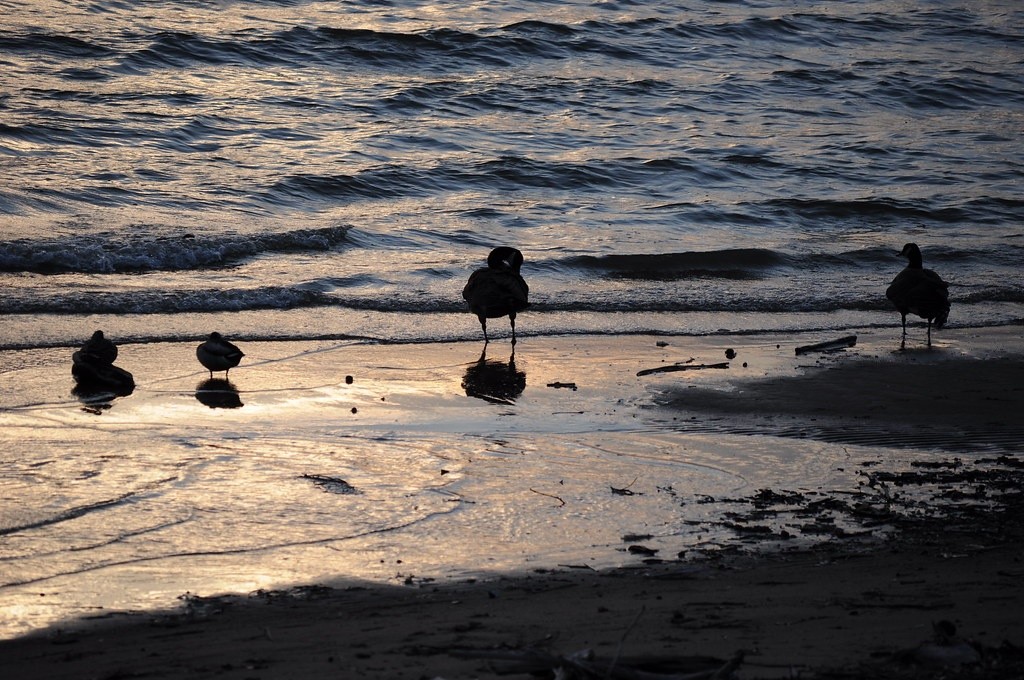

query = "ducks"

[
  {"left": 460, "top": 245, "right": 530, "bottom": 346},
  {"left": 884, "top": 241, "right": 953, "bottom": 349}
]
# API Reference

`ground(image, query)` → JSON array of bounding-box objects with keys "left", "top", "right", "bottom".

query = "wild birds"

[
  {"left": 72, "top": 329, "right": 119, "bottom": 368},
  {"left": 196, "top": 332, "right": 246, "bottom": 379}
]
